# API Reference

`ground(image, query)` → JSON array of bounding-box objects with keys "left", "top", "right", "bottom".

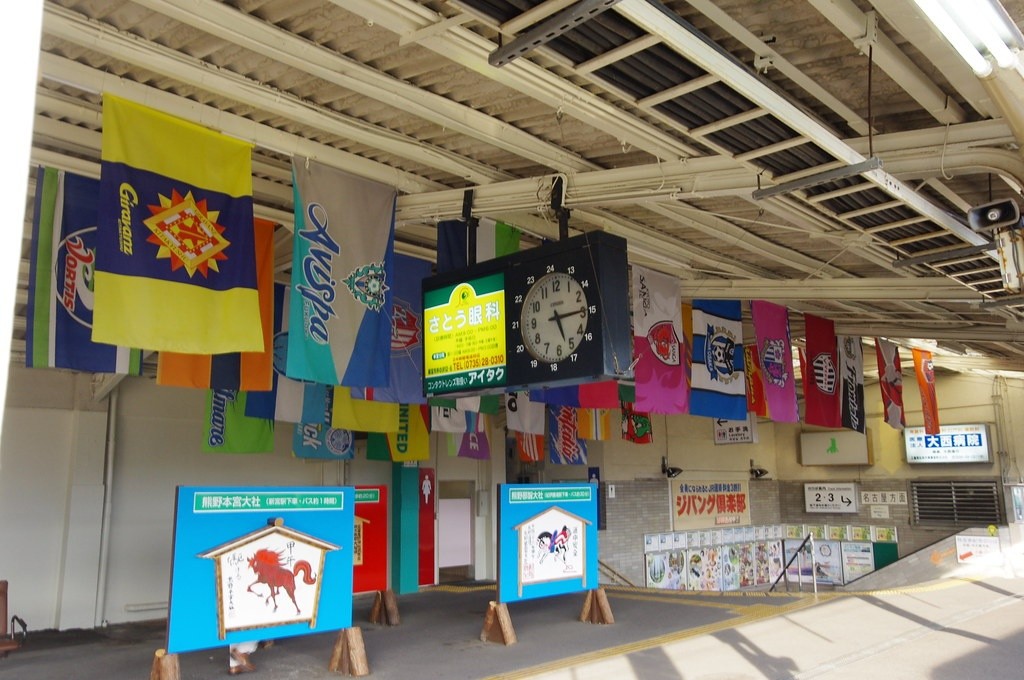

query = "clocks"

[{"left": 520, "top": 272, "right": 588, "bottom": 364}]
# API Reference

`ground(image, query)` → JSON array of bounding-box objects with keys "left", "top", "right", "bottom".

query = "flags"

[
  {"left": 201, "top": 250, "right": 652, "bottom": 466},
  {"left": 744, "top": 344, "right": 769, "bottom": 417},
  {"left": 875, "top": 337, "right": 906, "bottom": 429},
  {"left": 437, "top": 215, "right": 520, "bottom": 274},
  {"left": 153, "top": 216, "right": 273, "bottom": 390},
  {"left": 90, "top": 94, "right": 266, "bottom": 355},
  {"left": 284, "top": 156, "right": 395, "bottom": 386},
  {"left": 689, "top": 298, "right": 747, "bottom": 421},
  {"left": 804, "top": 312, "right": 842, "bottom": 428},
  {"left": 750, "top": 299, "right": 799, "bottom": 424},
  {"left": 913, "top": 349, "right": 940, "bottom": 434},
  {"left": 26, "top": 166, "right": 142, "bottom": 379},
  {"left": 633, "top": 264, "right": 688, "bottom": 415},
  {"left": 835, "top": 334, "right": 865, "bottom": 433},
  {"left": 797, "top": 348, "right": 805, "bottom": 398}
]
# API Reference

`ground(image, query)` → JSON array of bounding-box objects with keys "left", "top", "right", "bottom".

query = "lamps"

[
  {"left": 749, "top": 459, "right": 768, "bottom": 478},
  {"left": 661, "top": 455, "right": 684, "bottom": 478}
]
